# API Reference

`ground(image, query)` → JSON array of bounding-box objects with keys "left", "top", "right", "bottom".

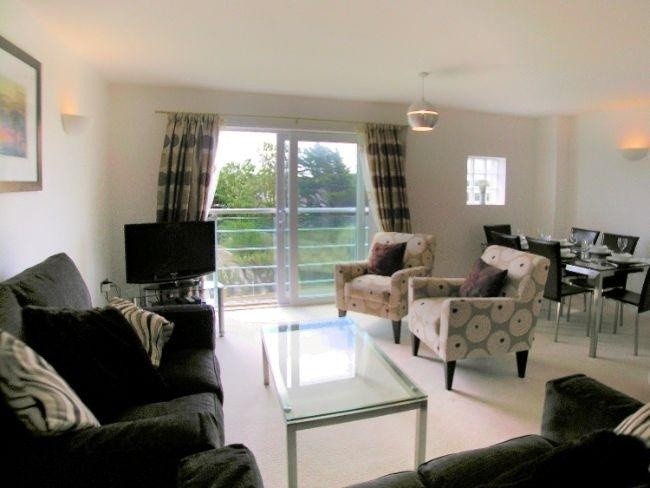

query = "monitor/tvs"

[{"left": 124, "top": 220, "right": 215, "bottom": 289}]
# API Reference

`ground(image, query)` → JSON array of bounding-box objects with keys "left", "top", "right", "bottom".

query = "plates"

[
  {"left": 608, "top": 256, "right": 641, "bottom": 264},
  {"left": 560, "top": 252, "right": 575, "bottom": 258},
  {"left": 561, "top": 243, "right": 573, "bottom": 247},
  {"left": 584, "top": 260, "right": 618, "bottom": 271},
  {"left": 588, "top": 248, "right": 612, "bottom": 256}
]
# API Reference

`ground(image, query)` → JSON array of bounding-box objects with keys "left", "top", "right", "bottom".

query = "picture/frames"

[{"left": 0, "top": 37, "right": 42, "bottom": 193}]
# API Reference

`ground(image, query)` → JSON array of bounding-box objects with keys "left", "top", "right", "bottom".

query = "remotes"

[
  {"left": 191, "top": 295, "right": 201, "bottom": 303},
  {"left": 184, "top": 295, "right": 195, "bottom": 304}
]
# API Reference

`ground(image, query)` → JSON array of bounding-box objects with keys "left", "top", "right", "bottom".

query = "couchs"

[
  {"left": 0, "top": 252, "right": 263, "bottom": 487},
  {"left": 344, "top": 373, "right": 650, "bottom": 488}
]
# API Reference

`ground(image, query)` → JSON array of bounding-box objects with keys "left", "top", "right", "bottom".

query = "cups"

[
  {"left": 560, "top": 248, "right": 570, "bottom": 255},
  {"left": 560, "top": 239, "right": 567, "bottom": 245},
  {"left": 581, "top": 251, "right": 589, "bottom": 260},
  {"left": 582, "top": 240, "right": 588, "bottom": 249}
]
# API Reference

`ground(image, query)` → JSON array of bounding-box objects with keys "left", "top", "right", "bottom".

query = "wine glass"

[{"left": 617, "top": 237, "right": 628, "bottom": 253}]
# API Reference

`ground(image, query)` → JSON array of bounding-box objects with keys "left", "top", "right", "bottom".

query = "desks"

[
  {"left": 127, "top": 280, "right": 226, "bottom": 337},
  {"left": 258, "top": 316, "right": 428, "bottom": 488}
]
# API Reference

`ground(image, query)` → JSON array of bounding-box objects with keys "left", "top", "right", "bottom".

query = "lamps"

[{"left": 406, "top": 71, "right": 439, "bottom": 131}]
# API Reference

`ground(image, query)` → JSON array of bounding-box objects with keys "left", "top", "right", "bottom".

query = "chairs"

[
  {"left": 335, "top": 231, "right": 435, "bottom": 343},
  {"left": 483, "top": 224, "right": 650, "bottom": 358},
  {"left": 407, "top": 245, "right": 551, "bottom": 391}
]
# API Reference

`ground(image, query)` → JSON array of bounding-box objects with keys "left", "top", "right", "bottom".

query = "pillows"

[
  {"left": 1, "top": 330, "right": 100, "bottom": 434},
  {"left": 110, "top": 297, "right": 175, "bottom": 369},
  {"left": 487, "top": 430, "right": 649, "bottom": 488},
  {"left": 25, "top": 306, "right": 165, "bottom": 419},
  {"left": 367, "top": 242, "right": 407, "bottom": 275},
  {"left": 457, "top": 258, "right": 507, "bottom": 297}
]
